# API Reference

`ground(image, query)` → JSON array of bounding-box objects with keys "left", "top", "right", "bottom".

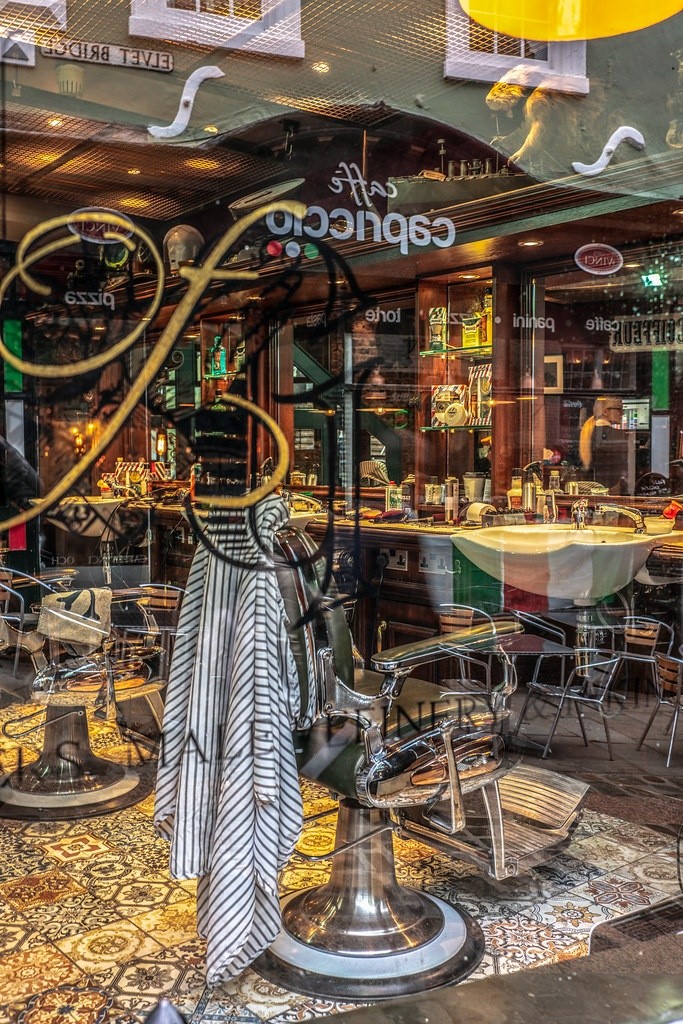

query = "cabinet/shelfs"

[
  {"left": 200, "top": 316, "right": 267, "bottom": 503},
  {"left": 416, "top": 263, "right": 522, "bottom": 523}
]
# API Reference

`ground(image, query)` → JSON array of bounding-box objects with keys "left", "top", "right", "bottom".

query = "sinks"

[{"left": 450, "top": 522, "right": 655, "bottom": 600}]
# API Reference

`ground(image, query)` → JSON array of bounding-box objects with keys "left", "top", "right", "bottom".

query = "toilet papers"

[{"left": 467, "top": 502, "right": 497, "bottom": 521}]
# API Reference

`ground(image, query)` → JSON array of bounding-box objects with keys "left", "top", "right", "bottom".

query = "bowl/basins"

[{"left": 644, "top": 517, "right": 675, "bottom": 535}]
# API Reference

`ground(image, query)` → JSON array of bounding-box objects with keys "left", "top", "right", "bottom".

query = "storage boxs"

[
  {"left": 461, "top": 318, "right": 482, "bottom": 353},
  {"left": 430, "top": 364, "right": 493, "bottom": 426}
]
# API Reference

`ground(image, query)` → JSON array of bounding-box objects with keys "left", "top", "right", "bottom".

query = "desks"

[{"left": 449, "top": 633, "right": 576, "bottom": 752}]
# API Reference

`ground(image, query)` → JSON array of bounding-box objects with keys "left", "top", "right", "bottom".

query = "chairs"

[
  {"left": 424, "top": 588, "right": 683, "bottom": 768},
  {"left": 0, "top": 562, "right": 197, "bottom": 819},
  {"left": 200, "top": 525, "right": 525, "bottom": 1000}
]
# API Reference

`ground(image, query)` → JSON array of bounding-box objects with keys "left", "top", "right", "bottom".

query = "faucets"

[{"left": 595, "top": 501, "right": 646, "bottom": 534}]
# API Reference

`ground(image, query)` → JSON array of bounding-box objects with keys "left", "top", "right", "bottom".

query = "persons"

[
  {"left": 579, "top": 396, "right": 627, "bottom": 490},
  {"left": 162, "top": 225, "right": 205, "bottom": 271}
]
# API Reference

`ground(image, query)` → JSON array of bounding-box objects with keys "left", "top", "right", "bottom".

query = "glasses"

[{"left": 606, "top": 406, "right": 624, "bottom": 415}]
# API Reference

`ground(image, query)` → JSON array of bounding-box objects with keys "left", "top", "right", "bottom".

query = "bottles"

[
  {"left": 292, "top": 466, "right": 301, "bottom": 474},
  {"left": 307, "top": 469, "right": 318, "bottom": 486}
]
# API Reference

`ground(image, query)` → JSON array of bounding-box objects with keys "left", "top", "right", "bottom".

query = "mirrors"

[{"left": 527, "top": 247, "right": 683, "bottom": 498}]
